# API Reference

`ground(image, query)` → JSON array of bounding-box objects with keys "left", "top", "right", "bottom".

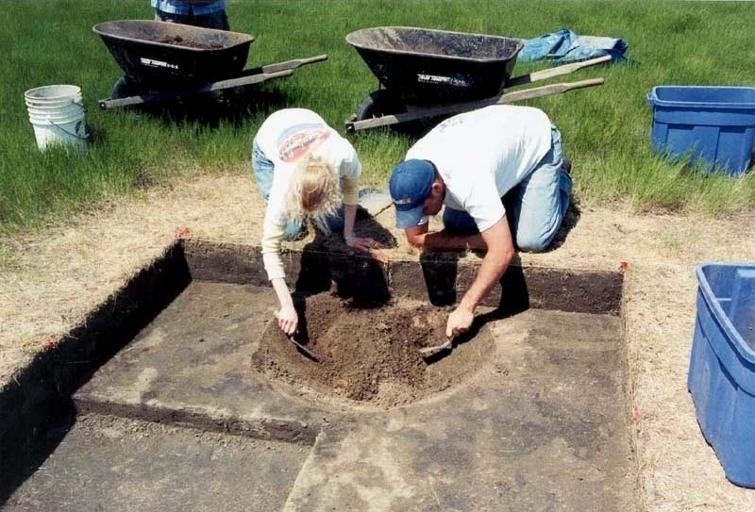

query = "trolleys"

[
  {"left": 90, "top": 14, "right": 332, "bottom": 113},
  {"left": 339, "top": 21, "right": 615, "bottom": 135}
]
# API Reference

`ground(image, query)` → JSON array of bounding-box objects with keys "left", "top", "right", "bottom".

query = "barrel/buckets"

[{"left": 25, "top": 84, "right": 88, "bottom": 154}]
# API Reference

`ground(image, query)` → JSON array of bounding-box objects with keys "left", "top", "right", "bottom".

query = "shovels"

[{"left": 273, "top": 309, "right": 321, "bottom": 361}]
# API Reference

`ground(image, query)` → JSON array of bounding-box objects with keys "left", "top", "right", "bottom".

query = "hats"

[{"left": 389, "top": 159, "right": 436, "bottom": 229}]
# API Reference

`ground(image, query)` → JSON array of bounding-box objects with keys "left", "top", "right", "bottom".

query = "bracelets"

[{"left": 345, "top": 232, "right": 355, "bottom": 240}]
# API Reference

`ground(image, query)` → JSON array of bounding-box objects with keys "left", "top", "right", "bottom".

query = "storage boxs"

[
  {"left": 687, "top": 262, "right": 755, "bottom": 490},
  {"left": 645, "top": 82, "right": 755, "bottom": 176}
]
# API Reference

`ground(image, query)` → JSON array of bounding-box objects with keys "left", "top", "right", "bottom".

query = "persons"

[
  {"left": 250, "top": 106, "right": 378, "bottom": 338},
  {"left": 388, "top": 102, "right": 574, "bottom": 338}
]
